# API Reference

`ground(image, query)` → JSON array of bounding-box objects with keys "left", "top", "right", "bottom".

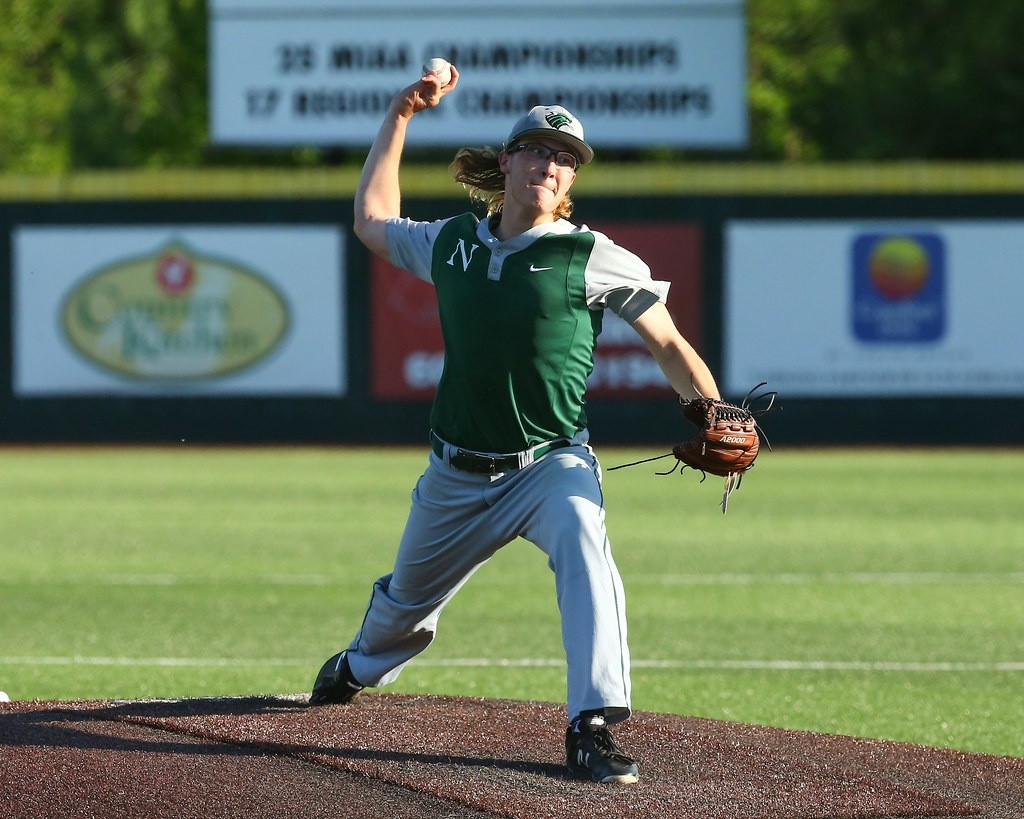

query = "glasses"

[{"left": 505, "top": 143, "right": 579, "bottom": 173}]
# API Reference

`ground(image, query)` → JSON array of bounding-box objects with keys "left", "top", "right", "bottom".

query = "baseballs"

[{"left": 422, "top": 57, "right": 452, "bottom": 88}]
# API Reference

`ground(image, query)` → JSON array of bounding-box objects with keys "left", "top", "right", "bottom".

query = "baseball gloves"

[{"left": 672, "top": 398, "right": 760, "bottom": 478}]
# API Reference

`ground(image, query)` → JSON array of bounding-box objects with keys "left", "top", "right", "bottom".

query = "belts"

[{"left": 430, "top": 435, "right": 569, "bottom": 475}]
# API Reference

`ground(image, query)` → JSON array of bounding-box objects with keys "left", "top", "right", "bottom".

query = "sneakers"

[
  {"left": 564, "top": 718, "right": 639, "bottom": 786},
  {"left": 310, "top": 647, "right": 366, "bottom": 705}
]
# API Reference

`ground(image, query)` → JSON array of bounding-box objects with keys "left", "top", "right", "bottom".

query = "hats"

[{"left": 507, "top": 104, "right": 594, "bottom": 166}]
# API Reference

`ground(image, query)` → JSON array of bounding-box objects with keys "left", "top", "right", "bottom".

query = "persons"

[{"left": 309, "top": 66, "right": 760, "bottom": 785}]
